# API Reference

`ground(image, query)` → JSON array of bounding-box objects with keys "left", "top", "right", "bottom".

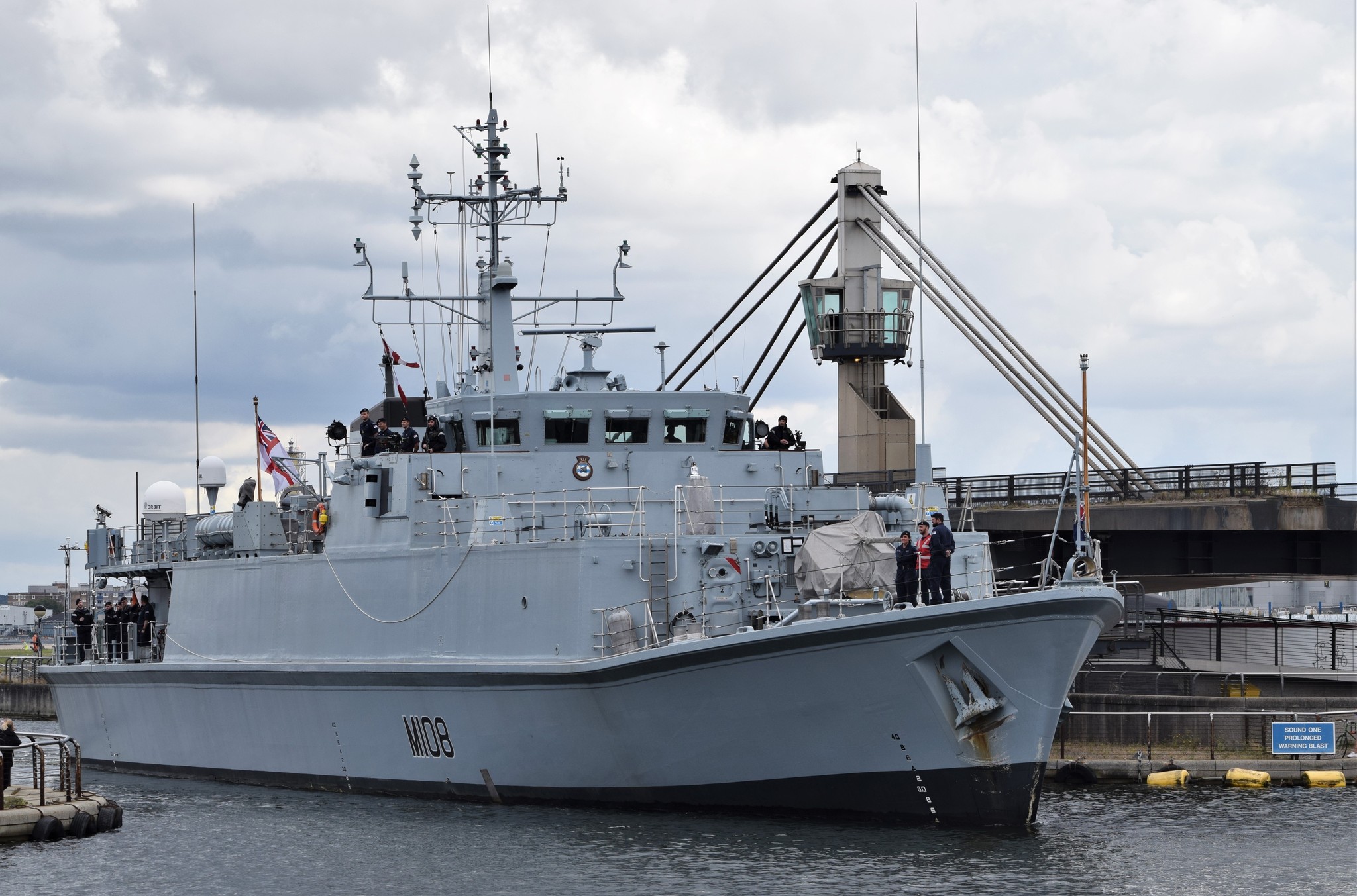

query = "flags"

[
  {"left": 382, "top": 338, "right": 420, "bottom": 368},
  {"left": 254, "top": 405, "right": 301, "bottom": 497},
  {"left": 391, "top": 369, "right": 408, "bottom": 406},
  {"left": 1073, "top": 501, "right": 1085, "bottom": 550}
]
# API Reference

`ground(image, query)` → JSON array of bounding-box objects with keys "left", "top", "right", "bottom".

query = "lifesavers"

[
  {"left": 33, "top": 817, "right": 64, "bottom": 841},
  {"left": 114, "top": 806, "right": 122, "bottom": 828},
  {"left": 1053, "top": 761, "right": 1097, "bottom": 785},
  {"left": 69, "top": 813, "right": 97, "bottom": 838},
  {"left": 97, "top": 807, "right": 117, "bottom": 831},
  {"left": 312, "top": 503, "right": 325, "bottom": 535},
  {"left": 1157, "top": 765, "right": 1192, "bottom": 778},
  {"left": 33, "top": 635, "right": 38, "bottom": 651}
]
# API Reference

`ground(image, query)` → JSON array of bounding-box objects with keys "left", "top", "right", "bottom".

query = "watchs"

[{"left": 142, "top": 628, "right": 145, "bottom": 631}]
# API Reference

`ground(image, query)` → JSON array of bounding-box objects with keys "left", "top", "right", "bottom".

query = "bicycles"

[{"left": 1333, "top": 718, "right": 1357, "bottom": 758}]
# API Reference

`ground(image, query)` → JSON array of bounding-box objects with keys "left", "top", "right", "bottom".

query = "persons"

[
  {"left": 22, "top": 640, "right": 28, "bottom": 650},
  {"left": 0, "top": 719, "right": 22, "bottom": 804},
  {"left": 913, "top": 521, "right": 931, "bottom": 608},
  {"left": 927, "top": 513, "right": 955, "bottom": 606},
  {"left": 360, "top": 408, "right": 379, "bottom": 456},
  {"left": 364, "top": 418, "right": 394, "bottom": 456},
  {"left": 421, "top": 416, "right": 447, "bottom": 453},
  {"left": 103, "top": 595, "right": 156, "bottom": 663},
  {"left": 761, "top": 415, "right": 796, "bottom": 450},
  {"left": 894, "top": 531, "right": 918, "bottom": 610},
  {"left": 71, "top": 598, "right": 97, "bottom": 663},
  {"left": 401, "top": 416, "right": 420, "bottom": 452}
]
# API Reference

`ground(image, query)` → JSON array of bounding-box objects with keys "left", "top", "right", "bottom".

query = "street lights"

[{"left": 33, "top": 605, "right": 47, "bottom": 657}]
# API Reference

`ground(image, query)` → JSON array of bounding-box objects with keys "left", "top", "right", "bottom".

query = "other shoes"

[{"left": 78, "top": 660, "right": 82, "bottom": 664}]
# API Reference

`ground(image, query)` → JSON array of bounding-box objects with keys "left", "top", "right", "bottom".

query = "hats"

[
  {"left": 130, "top": 598, "right": 132, "bottom": 602},
  {"left": 22, "top": 640, "right": 23, "bottom": 642},
  {"left": 778, "top": 415, "right": 788, "bottom": 421},
  {"left": 901, "top": 531, "right": 911, "bottom": 538},
  {"left": 427, "top": 416, "right": 437, "bottom": 424},
  {"left": 401, "top": 416, "right": 410, "bottom": 422},
  {"left": 918, "top": 521, "right": 929, "bottom": 525},
  {"left": 76, "top": 599, "right": 82, "bottom": 605},
  {"left": 105, "top": 601, "right": 112, "bottom": 606},
  {"left": 120, "top": 597, "right": 127, "bottom": 602},
  {"left": 141, "top": 595, "right": 149, "bottom": 601},
  {"left": 115, "top": 602, "right": 122, "bottom": 606},
  {"left": 931, "top": 512, "right": 943, "bottom": 517},
  {"left": 360, "top": 408, "right": 369, "bottom": 414},
  {"left": 376, "top": 418, "right": 386, "bottom": 424}
]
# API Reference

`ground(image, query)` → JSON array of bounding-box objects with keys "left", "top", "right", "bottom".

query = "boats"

[{"left": 34, "top": 1, "right": 1143, "bottom": 837}]
